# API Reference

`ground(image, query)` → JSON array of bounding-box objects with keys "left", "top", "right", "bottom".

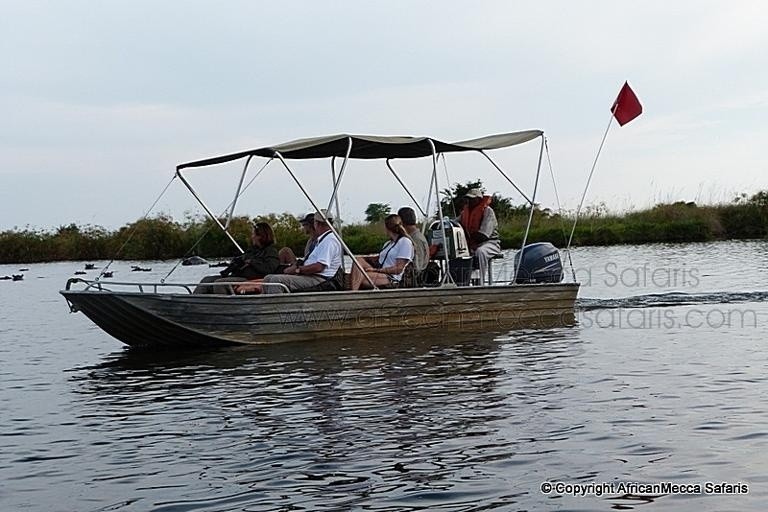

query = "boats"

[{"left": 58, "top": 130, "right": 582, "bottom": 351}]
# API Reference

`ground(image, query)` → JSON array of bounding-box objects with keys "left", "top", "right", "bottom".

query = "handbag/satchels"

[
  {"left": 220, "top": 256, "right": 245, "bottom": 277},
  {"left": 365, "top": 256, "right": 382, "bottom": 268}
]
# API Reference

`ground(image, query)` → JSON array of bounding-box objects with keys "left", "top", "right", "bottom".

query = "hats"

[
  {"left": 313, "top": 209, "right": 332, "bottom": 222},
  {"left": 464, "top": 188, "right": 483, "bottom": 198},
  {"left": 299, "top": 214, "right": 314, "bottom": 222}
]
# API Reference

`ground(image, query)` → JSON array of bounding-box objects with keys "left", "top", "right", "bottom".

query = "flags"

[{"left": 609, "top": 82, "right": 642, "bottom": 128}]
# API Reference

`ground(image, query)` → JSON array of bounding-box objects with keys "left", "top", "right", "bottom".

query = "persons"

[
  {"left": 453, "top": 187, "right": 500, "bottom": 285},
  {"left": 397, "top": 207, "right": 431, "bottom": 273},
  {"left": 193, "top": 221, "right": 278, "bottom": 295},
  {"left": 350, "top": 214, "right": 415, "bottom": 289},
  {"left": 279, "top": 212, "right": 318, "bottom": 263},
  {"left": 260, "top": 209, "right": 342, "bottom": 295}
]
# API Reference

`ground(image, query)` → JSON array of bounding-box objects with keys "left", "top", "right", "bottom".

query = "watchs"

[{"left": 295, "top": 264, "right": 301, "bottom": 274}]
